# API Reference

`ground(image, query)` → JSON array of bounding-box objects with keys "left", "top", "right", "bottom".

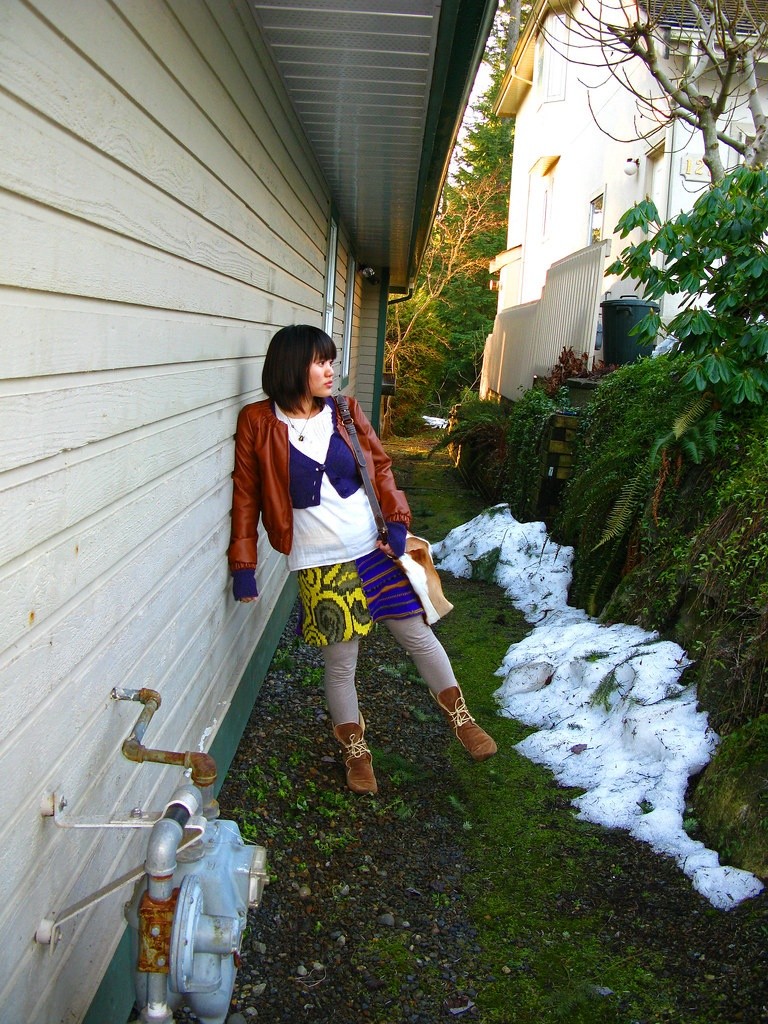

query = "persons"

[{"left": 230, "top": 325, "right": 497, "bottom": 793}]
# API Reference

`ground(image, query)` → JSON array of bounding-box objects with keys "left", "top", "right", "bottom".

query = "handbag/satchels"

[{"left": 383, "top": 527, "right": 453, "bottom": 625}]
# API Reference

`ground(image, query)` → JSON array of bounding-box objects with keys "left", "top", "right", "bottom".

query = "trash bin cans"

[{"left": 599, "top": 294, "right": 661, "bottom": 367}]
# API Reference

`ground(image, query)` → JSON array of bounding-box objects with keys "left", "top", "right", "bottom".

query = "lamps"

[
  {"left": 359, "top": 263, "right": 379, "bottom": 285},
  {"left": 624, "top": 157, "right": 640, "bottom": 176}
]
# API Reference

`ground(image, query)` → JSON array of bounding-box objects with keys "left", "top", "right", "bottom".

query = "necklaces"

[{"left": 287, "top": 408, "right": 311, "bottom": 441}]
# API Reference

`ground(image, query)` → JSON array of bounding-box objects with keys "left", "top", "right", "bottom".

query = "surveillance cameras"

[{"left": 369, "top": 275, "right": 380, "bottom": 286}]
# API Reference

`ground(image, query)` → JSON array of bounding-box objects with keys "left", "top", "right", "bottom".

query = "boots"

[
  {"left": 332, "top": 710, "right": 377, "bottom": 794},
  {"left": 429, "top": 680, "right": 497, "bottom": 761}
]
